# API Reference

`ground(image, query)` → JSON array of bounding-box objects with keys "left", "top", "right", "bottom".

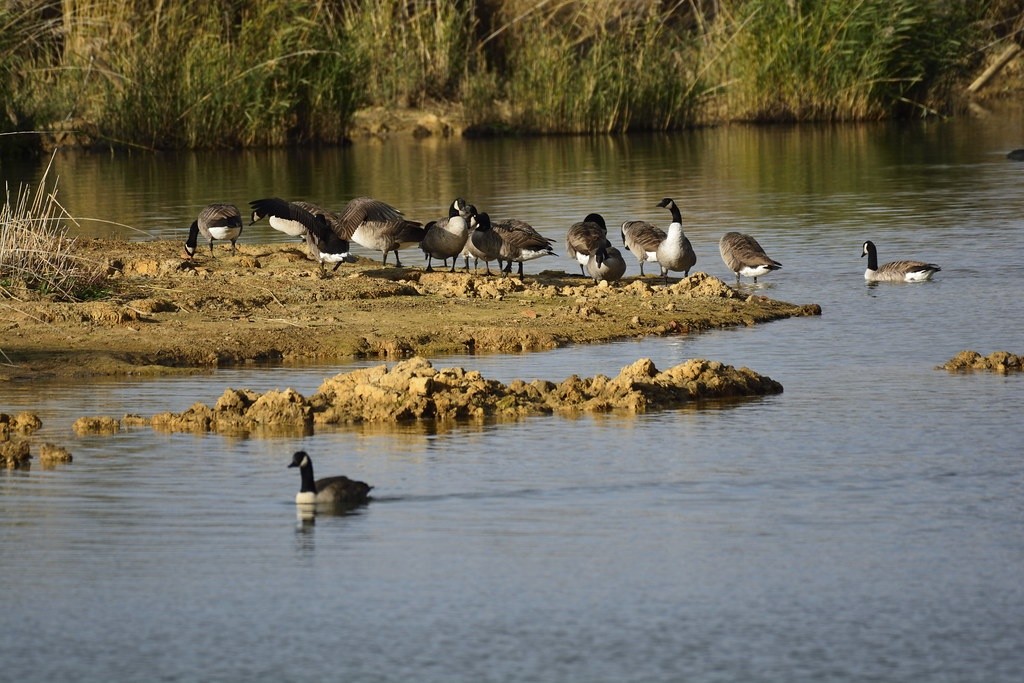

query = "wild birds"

[
  {"left": 719, "top": 232, "right": 783, "bottom": 284},
  {"left": 565, "top": 198, "right": 697, "bottom": 289},
  {"left": 286, "top": 451, "right": 376, "bottom": 506},
  {"left": 182, "top": 196, "right": 559, "bottom": 283},
  {"left": 860, "top": 241, "right": 943, "bottom": 283}
]
